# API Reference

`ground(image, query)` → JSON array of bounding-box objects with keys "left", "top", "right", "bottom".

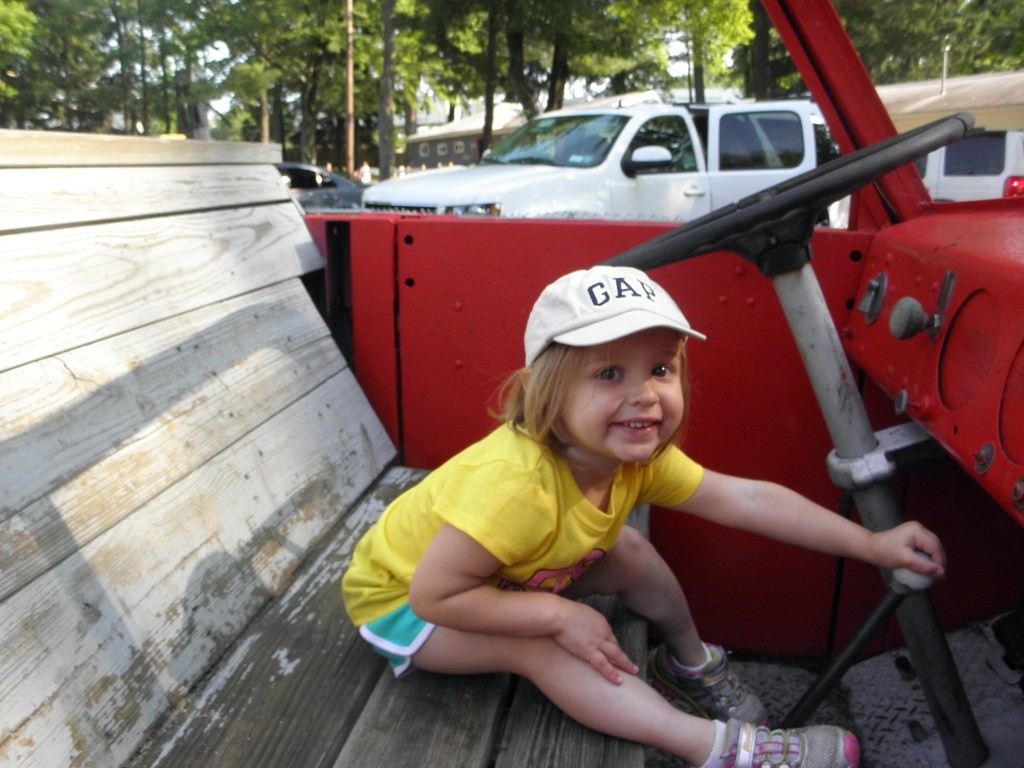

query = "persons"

[{"left": 343, "top": 263, "right": 944, "bottom": 768}]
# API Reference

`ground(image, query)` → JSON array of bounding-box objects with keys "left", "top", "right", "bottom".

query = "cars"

[
  {"left": 361, "top": 99, "right": 851, "bottom": 230},
  {"left": 276, "top": 164, "right": 365, "bottom": 213},
  {"left": 913, "top": 128, "right": 1024, "bottom": 197}
]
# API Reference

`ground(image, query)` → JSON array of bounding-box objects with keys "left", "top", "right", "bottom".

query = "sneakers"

[
  {"left": 647, "top": 641, "right": 768, "bottom": 732},
  {"left": 719, "top": 718, "right": 860, "bottom": 768}
]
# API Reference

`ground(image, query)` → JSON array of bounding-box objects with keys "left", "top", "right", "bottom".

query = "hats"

[{"left": 524, "top": 266, "right": 706, "bottom": 369}]
